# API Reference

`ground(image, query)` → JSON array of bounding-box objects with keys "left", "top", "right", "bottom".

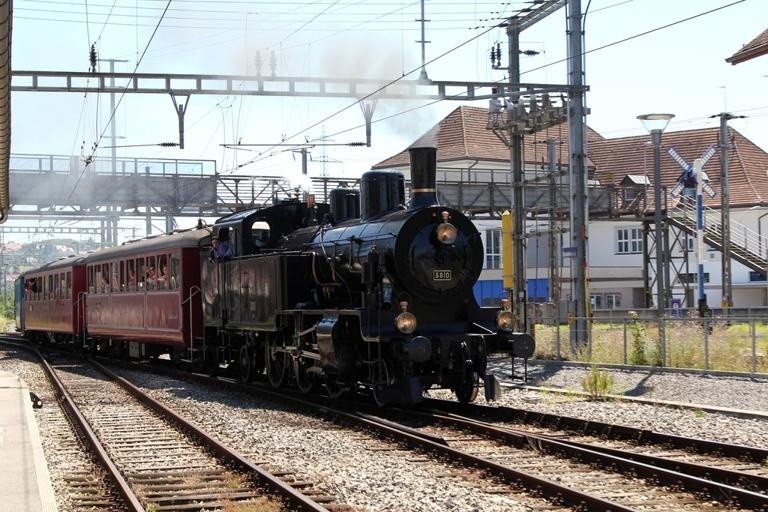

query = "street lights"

[{"left": 636, "top": 112, "right": 673, "bottom": 319}]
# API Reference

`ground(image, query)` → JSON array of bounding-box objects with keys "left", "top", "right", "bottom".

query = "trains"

[{"left": 13, "top": 147, "right": 534, "bottom": 409}]
